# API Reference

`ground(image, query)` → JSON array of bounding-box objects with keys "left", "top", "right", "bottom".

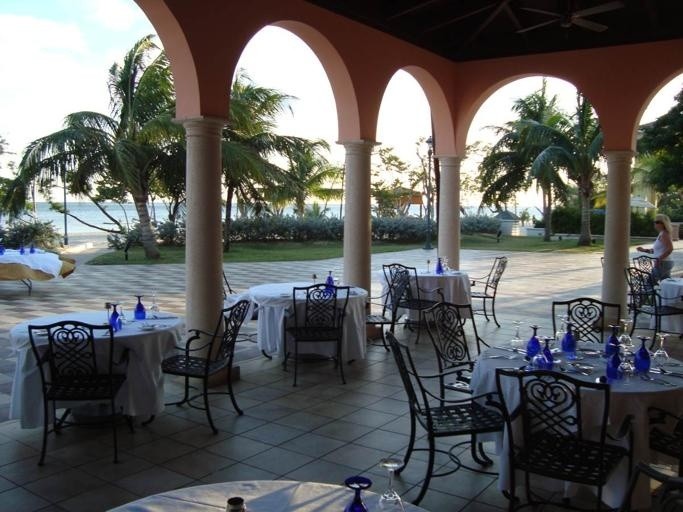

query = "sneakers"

[{"left": 627, "top": 303, "right": 638, "bottom": 309}]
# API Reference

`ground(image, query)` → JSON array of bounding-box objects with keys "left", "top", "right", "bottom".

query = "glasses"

[{"left": 654, "top": 221, "right": 662, "bottom": 223}]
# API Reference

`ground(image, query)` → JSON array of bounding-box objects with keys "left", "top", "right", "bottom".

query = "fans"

[{"left": 511, "top": 2, "right": 628, "bottom": 41}]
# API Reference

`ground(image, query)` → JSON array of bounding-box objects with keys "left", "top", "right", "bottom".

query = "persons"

[{"left": 636, "top": 213, "right": 675, "bottom": 287}]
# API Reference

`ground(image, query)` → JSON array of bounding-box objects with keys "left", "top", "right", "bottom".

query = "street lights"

[{"left": 423, "top": 134, "right": 433, "bottom": 250}]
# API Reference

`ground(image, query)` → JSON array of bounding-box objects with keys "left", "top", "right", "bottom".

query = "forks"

[
  {"left": 576, "top": 347, "right": 602, "bottom": 359},
  {"left": 637, "top": 367, "right": 682, "bottom": 388},
  {"left": 484, "top": 345, "right": 525, "bottom": 370},
  {"left": 560, "top": 363, "right": 595, "bottom": 376}
]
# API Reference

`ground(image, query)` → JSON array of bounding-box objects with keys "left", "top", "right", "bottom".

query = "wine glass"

[
  {"left": 633, "top": 336, "right": 650, "bottom": 372},
  {"left": 344, "top": 476, "right": 371, "bottom": 512},
  {"left": 560, "top": 322, "right": 575, "bottom": 359},
  {"left": 119, "top": 304, "right": 126, "bottom": 324},
  {"left": 511, "top": 321, "right": 524, "bottom": 347},
  {"left": 618, "top": 343, "right": 633, "bottom": 374},
  {"left": 555, "top": 315, "right": 568, "bottom": 347},
  {"left": 435, "top": 258, "right": 443, "bottom": 274},
  {"left": 19, "top": 243, "right": 25, "bottom": 255},
  {"left": 526, "top": 325, "right": 541, "bottom": 358},
  {"left": 606, "top": 344, "right": 622, "bottom": 380},
  {"left": 324, "top": 271, "right": 334, "bottom": 294},
  {"left": 603, "top": 326, "right": 619, "bottom": 358},
  {"left": 0, "top": 242, "right": 4, "bottom": 255},
  {"left": 133, "top": 295, "right": 146, "bottom": 319},
  {"left": 108, "top": 303, "right": 122, "bottom": 331},
  {"left": 538, "top": 338, "right": 554, "bottom": 370},
  {"left": 148, "top": 295, "right": 159, "bottom": 318},
  {"left": 618, "top": 318, "right": 632, "bottom": 347},
  {"left": 29, "top": 243, "right": 34, "bottom": 254},
  {"left": 375, "top": 458, "right": 407, "bottom": 512},
  {"left": 652, "top": 334, "right": 669, "bottom": 366}
]
